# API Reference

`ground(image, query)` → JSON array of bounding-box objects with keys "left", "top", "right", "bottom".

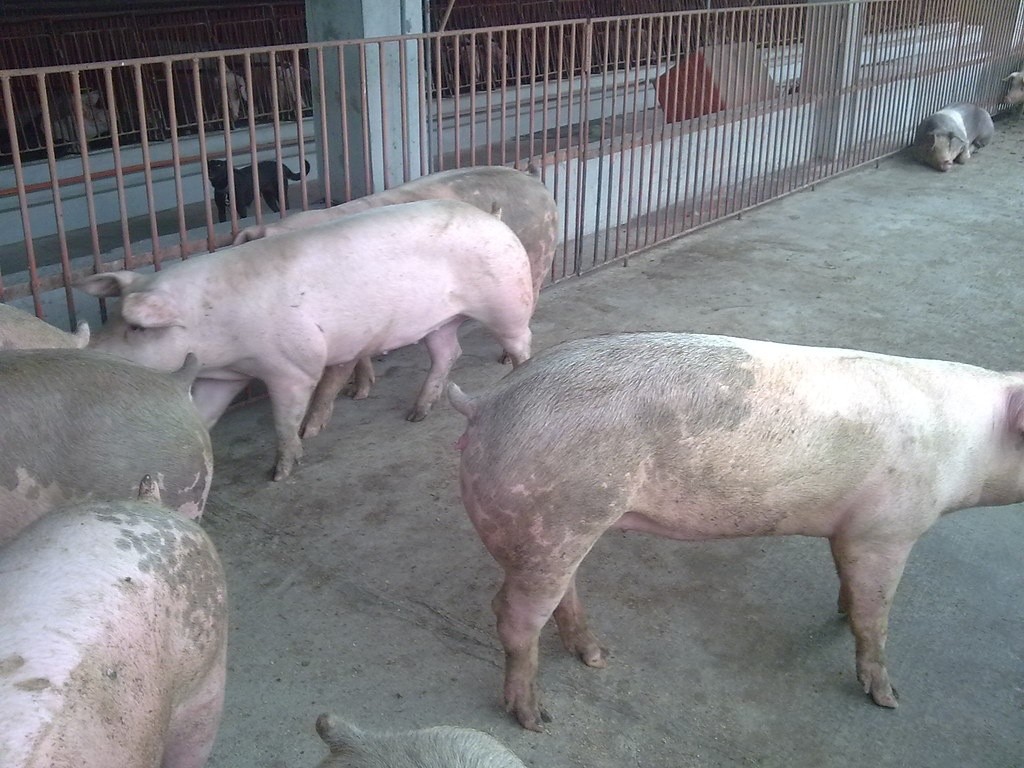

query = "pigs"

[
  {"left": 0, "top": 159, "right": 563, "bottom": 767},
  {"left": 447, "top": 329, "right": 1024, "bottom": 730},
  {"left": 255, "top": 59, "right": 311, "bottom": 110},
  {"left": 1002, "top": 69, "right": 1024, "bottom": 107},
  {"left": 188, "top": 69, "right": 249, "bottom": 120},
  {"left": 912, "top": 102, "right": 994, "bottom": 172}
]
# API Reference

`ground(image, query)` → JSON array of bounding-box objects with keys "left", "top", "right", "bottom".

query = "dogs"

[{"left": 207, "top": 155, "right": 311, "bottom": 222}]
